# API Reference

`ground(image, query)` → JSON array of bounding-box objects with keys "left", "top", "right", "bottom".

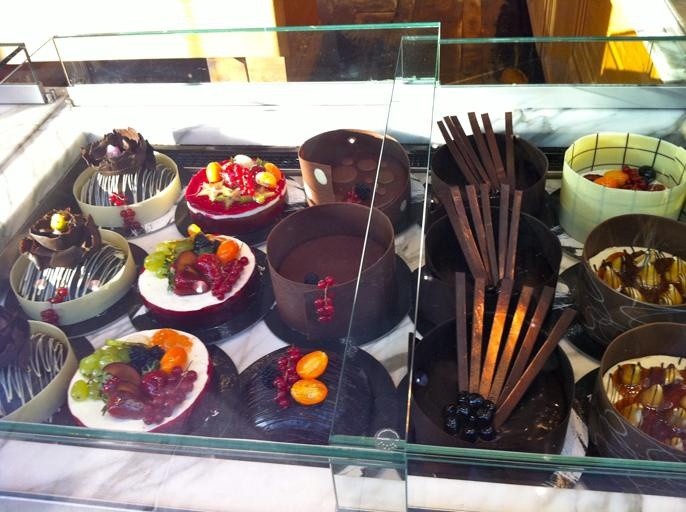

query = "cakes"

[
  {"left": 9, "top": 207, "right": 137, "bottom": 326},
  {"left": 425, "top": 183, "right": 562, "bottom": 326},
  {"left": 185, "top": 155, "right": 289, "bottom": 237},
  {"left": 430, "top": 113, "right": 547, "bottom": 215},
  {"left": 71, "top": 126, "right": 181, "bottom": 228},
  {"left": 0, "top": 308, "right": 79, "bottom": 424},
  {"left": 236, "top": 343, "right": 373, "bottom": 445},
  {"left": 67, "top": 327, "right": 216, "bottom": 435},
  {"left": 137, "top": 223, "right": 262, "bottom": 331},
  {"left": 297, "top": 128, "right": 411, "bottom": 227},
  {"left": 407, "top": 272, "right": 578, "bottom": 486},
  {"left": 266, "top": 202, "right": 396, "bottom": 339},
  {"left": 558, "top": 132, "right": 685, "bottom": 244},
  {"left": 579, "top": 214, "right": 685, "bottom": 347},
  {"left": 588, "top": 322, "right": 686, "bottom": 497}
]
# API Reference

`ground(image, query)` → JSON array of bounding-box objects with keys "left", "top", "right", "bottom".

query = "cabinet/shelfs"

[{"left": 0, "top": 21, "right": 686, "bottom": 511}]
